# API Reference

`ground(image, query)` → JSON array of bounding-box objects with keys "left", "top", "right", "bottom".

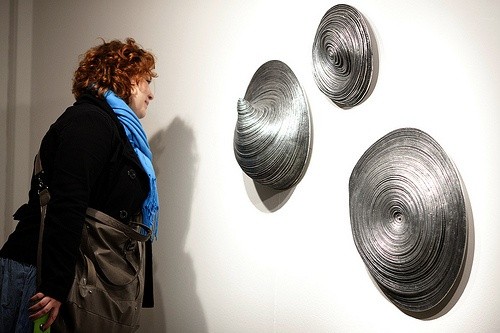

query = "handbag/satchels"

[{"left": 36, "top": 193, "right": 154, "bottom": 333}]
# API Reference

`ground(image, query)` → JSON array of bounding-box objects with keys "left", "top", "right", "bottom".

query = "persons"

[{"left": 0, "top": 31, "right": 158, "bottom": 332}]
